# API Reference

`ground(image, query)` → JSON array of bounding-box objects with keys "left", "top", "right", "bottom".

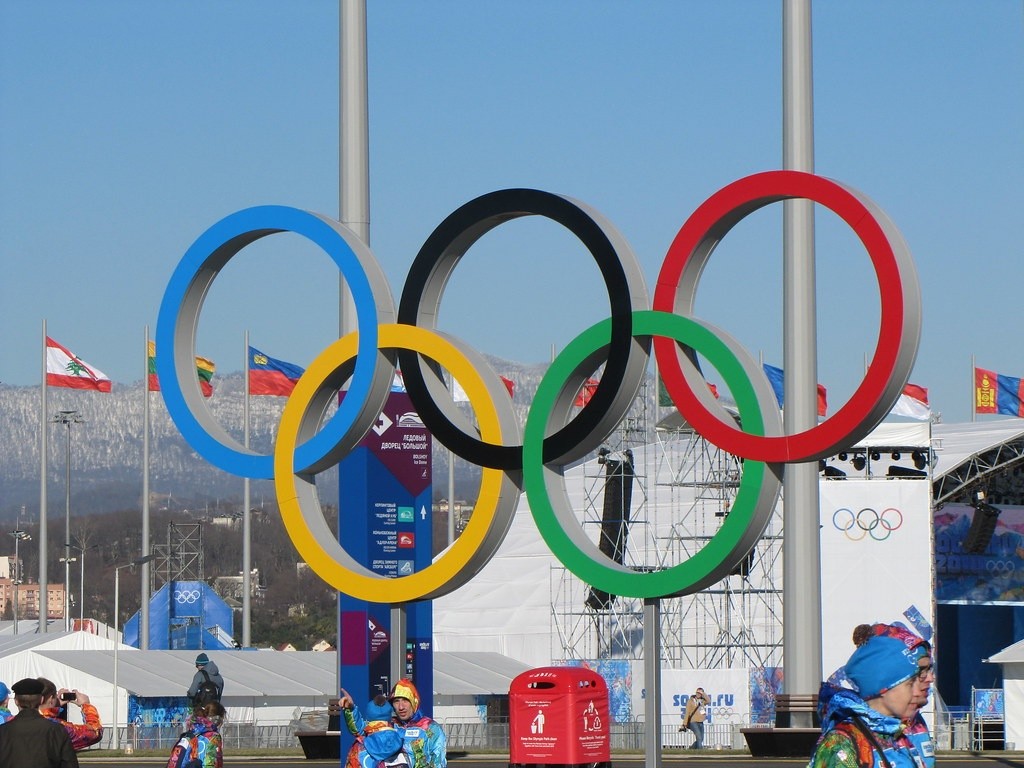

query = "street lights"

[
  {"left": 6, "top": 517, "right": 33, "bottom": 635},
  {"left": 64, "top": 544, "right": 102, "bottom": 631},
  {"left": 114, "top": 554, "right": 157, "bottom": 749},
  {"left": 49, "top": 410, "right": 86, "bottom": 633}
]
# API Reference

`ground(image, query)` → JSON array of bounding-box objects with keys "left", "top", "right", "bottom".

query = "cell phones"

[{"left": 64, "top": 692, "right": 77, "bottom": 699}]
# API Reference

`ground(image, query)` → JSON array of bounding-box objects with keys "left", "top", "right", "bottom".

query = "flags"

[
  {"left": 248, "top": 346, "right": 305, "bottom": 397},
  {"left": 148, "top": 340, "right": 215, "bottom": 397},
  {"left": 452, "top": 375, "right": 515, "bottom": 402},
  {"left": 888, "top": 383, "right": 930, "bottom": 420},
  {"left": 46, "top": 336, "right": 111, "bottom": 393},
  {"left": 390, "top": 369, "right": 407, "bottom": 393},
  {"left": 975, "top": 367, "right": 1024, "bottom": 418},
  {"left": 573, "top": 378, "right": 599, "bottom": 407},
  {"left": 763, "top": 363, "right": 828, "bottom": 416}
]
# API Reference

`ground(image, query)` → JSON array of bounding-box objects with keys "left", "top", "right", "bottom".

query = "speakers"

[
  {"left": 963, "top": 504, "right": 1000, "bottom": 554},
  {"left": 584, "top": 460, "right": 636, "bottom": 610},
  {"left": 733, "top": 548, "right": 756, "bottom": 576}
]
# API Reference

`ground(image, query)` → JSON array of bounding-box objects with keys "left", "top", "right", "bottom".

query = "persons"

[
  {"left": 338, "top": 676, "right": 448, "bottom": 768},
  {"left": 0, "top": 681, "right": 15, "bottom": 726},
  {"left": 165, "top": 703, "right": 227, "bottom": 768},
  {"left": 188, "top": 653, "right": 223, "bottom": 708},
  {"left": 683, "top": 688, "right": 711, "bottom": 749},
  {"left": 37, "top": 678, "right": 104, "bottom": 750},
  {"left": 0, "top": 679, "right": 79, "bottom": 768},
  {"left": 805, "top": 623, "right": 936, "bottom": 768}
]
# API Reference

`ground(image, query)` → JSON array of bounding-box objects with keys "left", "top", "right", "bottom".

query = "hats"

[
  {"left": 364, "top": 695, "right": 392, "bottom": 721},
  {"left": 0, "top": 682, "right": 11, "bottom": 702},
  {"left": 12, "top": 678, "right": 44, "bottom": 694},
  {"left": 910, "top": 644, "right": 929, "bottom": 660},
  {"left": 196, "top": 653, "right": 209, "bottom": 665},
  {"left": 846, "top": 624, "right": 919, "bottom": 700}
]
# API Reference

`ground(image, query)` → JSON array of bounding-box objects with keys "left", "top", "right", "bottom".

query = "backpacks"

[
  {"left": 377, "top": 748, "right": 412, "bottom": 768},
  {"left": 166, "top": 730, "right": 218, "bottom": 768},
  {"left": 193, "top": 669, "right": 221, "bottom": 708}
]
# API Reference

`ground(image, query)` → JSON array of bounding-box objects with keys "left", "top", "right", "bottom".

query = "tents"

[
  {"left": 0, "top": 650, "right": 538, "bottom": 747},
  {"left": 0, "top": 618, "right": 140, "bottom": 651}
]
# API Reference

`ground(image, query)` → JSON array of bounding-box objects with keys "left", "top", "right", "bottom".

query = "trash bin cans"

[{"left": 508, "top": 667, "right": 612, "bottom": 768}]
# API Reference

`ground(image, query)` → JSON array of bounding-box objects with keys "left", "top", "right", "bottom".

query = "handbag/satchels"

[{"left": 687, "top": 718, "right": 690, "bottom": 729}]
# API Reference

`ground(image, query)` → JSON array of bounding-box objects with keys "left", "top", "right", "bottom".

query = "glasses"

[
  {"left": 917, "top": 668, "right": 935, "bottom": 678},
  {"left": 196, "top": 665, "right": 204, "bottom": 668}
]
